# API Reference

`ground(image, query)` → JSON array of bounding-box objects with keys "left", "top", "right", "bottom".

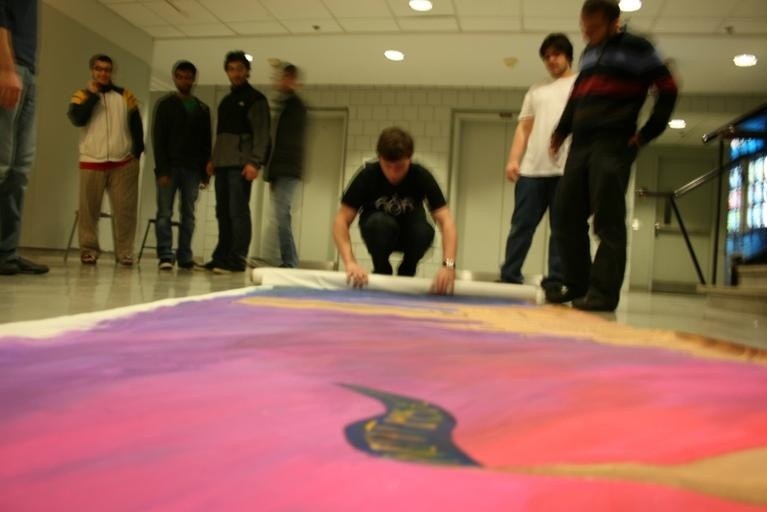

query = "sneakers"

[
  {"left": 212, "top": 266, "right": 235, "bottom": 274},
  {"left": 178, "top": 263, "right": 195, "bottom": 271},
  {"left": 159, "top": 260, "right": 173, "bottom": 271}
]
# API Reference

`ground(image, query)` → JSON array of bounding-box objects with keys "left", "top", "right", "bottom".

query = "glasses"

[{"left": 92, "top": 66, "right": 114, "bottom": 73}]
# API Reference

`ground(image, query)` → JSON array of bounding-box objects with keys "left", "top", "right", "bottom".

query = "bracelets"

[{"left": 441, "top": 258, "right": 456, "bottom": 269}]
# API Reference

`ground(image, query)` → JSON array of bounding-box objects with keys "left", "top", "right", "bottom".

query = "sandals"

[
  {"left": 115, "top": 255, "right": 133, "bottom": 266},
  {"left": 80, "top": 254, "right": 98, "bottom": 264}
]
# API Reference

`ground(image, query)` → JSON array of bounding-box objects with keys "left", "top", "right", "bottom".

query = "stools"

[{"left": 61, "top": 209, "right": 180, "bottom": 266}]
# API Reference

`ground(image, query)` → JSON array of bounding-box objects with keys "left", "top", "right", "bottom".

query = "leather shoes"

[
  {"left": 547, "top": 285, "right": 579, "bottom": 302},
  {"left": 0, "top": 260, "right": 18, "bottom": 275},
  {"left": 573, "top": 297, "right": 616, "bottom": 312},
  {"left": 14, "top": 257, "right": 50, "bottom": 275}
]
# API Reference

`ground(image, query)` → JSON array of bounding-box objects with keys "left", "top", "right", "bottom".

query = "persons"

[
  {"left": 543, "top": 0, "right": 680, "bottom": 316},
  {"left": 203, "top": 48, "right": 271, "bottom": 274},
  {"left": 330, "top": 125, "right": 460, "bottom": 298},
  {"left": 0, "top": 1, "right": 54, "bottom": 279},
  {"left": 66, "top": 53, "right": 146, "bottom": 268},
  {"left": 261, "top": 62, "right": 311, "bottom": 269},
  {"left": 495, "top": 29, "right": 582, "bottom": 287},
  {"left": 152, "top": 58, "right": 216, "bottom": 273}
]
